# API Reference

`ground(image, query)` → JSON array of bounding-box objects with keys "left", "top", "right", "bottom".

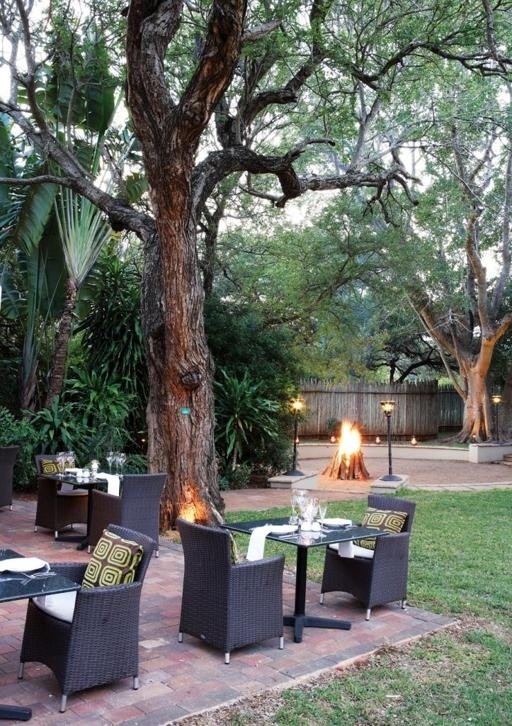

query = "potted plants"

[{"left": 0, "top": 404, "right": 47, "bottom": 510}]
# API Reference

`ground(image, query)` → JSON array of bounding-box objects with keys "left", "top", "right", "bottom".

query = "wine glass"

[
  {"left": 288, "top": 488, "right": 328, "bottom": 532},
  {"left": 105, "top": 452, "right": 127, "bottom": 479},
  {"left": 57, "top": 451, "right": 75, "bottom": 476}
]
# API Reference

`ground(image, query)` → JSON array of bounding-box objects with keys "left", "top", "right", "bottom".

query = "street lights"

[
  {"left": 380, "top": 399, "right": 401, "bottom": 482},
  {"left": 282, "top": 396, "right": 306, "bottom": 475},
  {"left": 493, "top": 385, "right": 505, "bottom": 444}
]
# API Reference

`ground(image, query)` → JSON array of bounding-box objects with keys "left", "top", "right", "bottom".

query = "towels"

[
  {"left": 247, "top": 524, "right": 299, "bottom": 561},
  {"left": 94, "top": 471, "right": 120, "bottom": 496},
  {"left": 0, "top": 557, "right": 50, "bottom": 573},
  {"left": 319, "top": 517, "right": 354, "bottom": 558}
]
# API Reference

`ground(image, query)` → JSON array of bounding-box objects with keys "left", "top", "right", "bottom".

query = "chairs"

[
  {"left": 18, "top": 523, "right": 158, "bottom": 712},
  {"left": 176, "top": 518, "right": 285, "bottom": 664},
  {"left": 91, "top": 474, "right": 168, "bottom": 558},
  {"left": 34, "top": 454, "right": 89, "bottom": 539},
  {"left": 319, "top": 494, "right": 417, "bottom": 620}
]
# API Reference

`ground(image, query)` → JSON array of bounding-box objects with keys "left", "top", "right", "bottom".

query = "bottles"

[
  {"left": 75, "top": 460, "right": 100, "bottom": 477},
  {"left": 76, "top": 477, "right": 97, "bottom": 483}
]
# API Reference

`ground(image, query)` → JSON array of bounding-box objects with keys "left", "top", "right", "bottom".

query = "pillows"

[
  {"left": 82, "top": 528, "right": 143, "bottom": 592},
  {"left": 40, "top": 458, "right": 71, "bottom": 474},
  {"left": 193, "top": 518, "right": 240, "bottom": 565},
  {"left": 353, "top": 506, "right": 408, "bottom": 550}
]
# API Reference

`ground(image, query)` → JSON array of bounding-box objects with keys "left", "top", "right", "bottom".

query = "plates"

[
  {"left": 0, "top": 557, "right": 46, "bottom": 575},
  {"left": 266, "top": 533, "right": 297, "bottom": 539},
  {"left": 323, "top": 526, "right": 352, "bottom": 534},
  {"left": 249, "top": 525, "right": 298, "bottom": 535},
  {"left": 318, "top": 519, "right": 350, "bottom": 528},
  {"left": 1, "top": 572, "right": 46, "bottom": 582}
]
